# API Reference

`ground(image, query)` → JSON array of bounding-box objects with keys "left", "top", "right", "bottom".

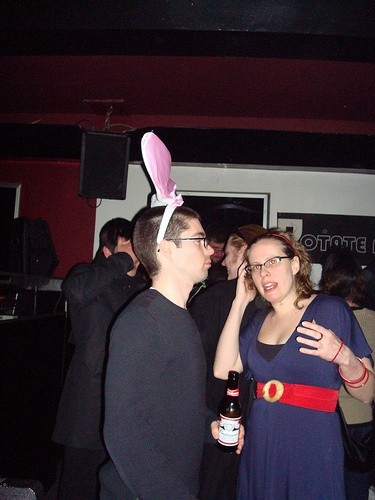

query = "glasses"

[
  {"left": 157, "top": 235, "right": 208, "bottom": 252},
  {"left": 244, "top": 255, "right": 291, "bottom": 276},
  {"left": 212, "top": 247, "right": 224, "bottom": 253}
]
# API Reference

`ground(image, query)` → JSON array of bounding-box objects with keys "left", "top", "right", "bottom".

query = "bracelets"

[
  {"left": 337, "top": 357, "right": 370, "bottom": 388},
  {"left": 329, "top": 339, "right": 344, "bottom": 362}
]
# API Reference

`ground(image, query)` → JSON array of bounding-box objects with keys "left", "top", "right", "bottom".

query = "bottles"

[{"left": 213, "top": 371, "right": 244, "bottom": 456}]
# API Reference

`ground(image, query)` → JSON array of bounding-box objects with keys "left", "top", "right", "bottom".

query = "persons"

[
  {"left": 213, "top": 227, "right": 375, "bottom": 500},
  {"left": 99, "top": 206, "right": 244, "bottom": 500},
  {"left": 188, "top": 224, "right": 269, "bottom": 356},
  {"left": 186, "top": 226, "right": 229, "bottom": 281},
  {"left": 318, "top": 248, "right": 375, "bottom": 500},
  {"left": 50, "top": 217, "right": 153, "bottom": 500}
]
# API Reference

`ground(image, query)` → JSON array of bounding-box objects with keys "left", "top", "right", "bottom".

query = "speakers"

[
  {"left": 77, "top": 130, "right": 130, "bottom": 200},
  {"left": 10, "top": 217, "right": 57, "bottom": 288}
]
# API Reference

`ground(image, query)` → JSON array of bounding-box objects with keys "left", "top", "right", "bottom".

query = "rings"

[{"left": 317, "top": 333, "right": 323, "bottom": 341}]
